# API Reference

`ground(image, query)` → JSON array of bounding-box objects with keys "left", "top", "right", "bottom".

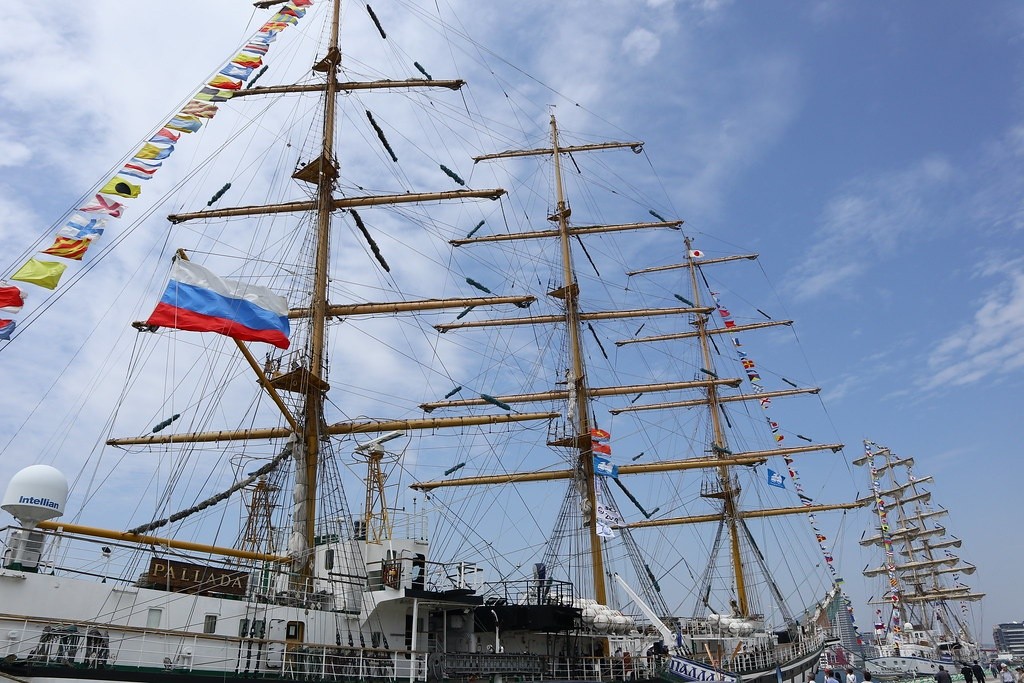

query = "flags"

[
  {"left": 165, "top": 114, "right": 203, "bottom": 133},
  {"left": 220, "top": 0, "right": 316, "bottom": 81},
  {"left": 863, "top": 438, "right": 968, "bottom": 634},
  {"left": 79, "top": 193, "right": 127, "bottom": 219},
  {"left": 0, "top": 279, "right": 28, "bottom": 342},
  {"left": 135, "top": 142, "right": 174, "bottom": 161},
  {"left": 207, "top": 74, "right": 243, "bottom": 93},
  {"left": 711, "top": 292, "right": 860, "bottom": 638},
  {"left": 180, "top": 100, "right": 218, "bottom": 119},
  {"left": 145, "top": 258, "right": 291, "bottom": 350},
  {"left": 149, "top": 127, "right": 181, "bottom": 145},
  {"left": 100, "top": 175, "right": 141, "bottom": 199},
  {"left": 118, "top": 157, "right": 162, "bottom": 181},
  {"left": 689, "top": 249, "right": 705, "bottom": 257},
  {"left": 588, "top": 427, "right": 620, "bottom": 539},
  {"left": 41, "top": 213, "right": 106, "bottom": 262},
  {"left": 193, "top": 86, "right": 233, "bottom": 107},
  {"left": 11, "top": 255, "right": 67, "bottom": 291}
]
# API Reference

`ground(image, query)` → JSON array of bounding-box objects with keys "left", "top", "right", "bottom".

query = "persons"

[
  {"left": 989, "top": 658, "right": 1024, "bottom": 683},
  {"left": 961, "top": 660, "right": 986, "bottom": 683},
  {"left": 934, "top": 665, "right": 952, "bottom": 683},
  {"left": 807, "top": 668, "right": 874, "bottom": 683}
]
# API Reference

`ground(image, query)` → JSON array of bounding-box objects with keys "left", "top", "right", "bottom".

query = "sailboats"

[{"left": 0, "top": 1, "right": 1024, "bottom": 683}]
